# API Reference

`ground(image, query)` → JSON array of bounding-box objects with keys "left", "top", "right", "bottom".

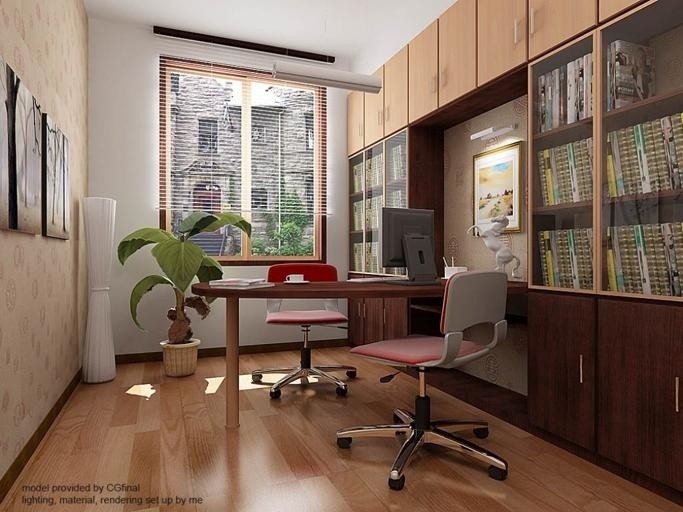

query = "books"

[
  {"left": 209, "top": 282, "right": 277, "bottom": 289},
  {"left": 208, "top": 277, "right": 265, "bottom": 286},
  {"left": 528, "top": 40, "right": 681, "bottom": 299},
  {"left": 350, "top": 143, "right": 406, "bottom": 275}
]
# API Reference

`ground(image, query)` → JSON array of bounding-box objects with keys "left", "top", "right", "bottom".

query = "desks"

[{"left": 192, "top": 271, "right": 527, "bottom": 430}]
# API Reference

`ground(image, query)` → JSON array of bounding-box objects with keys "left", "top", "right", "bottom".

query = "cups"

[{"left": 285, "top": 274, "right": 304, "bottom": 281}]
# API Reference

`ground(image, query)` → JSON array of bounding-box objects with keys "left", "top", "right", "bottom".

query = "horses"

[{"left": 466, "top": 214, "right": 522, "bottom": 279}]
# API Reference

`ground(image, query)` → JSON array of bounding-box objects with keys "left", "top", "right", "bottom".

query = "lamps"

[{"left": 271, "top": 0, "right": 382, "bottom": 94}]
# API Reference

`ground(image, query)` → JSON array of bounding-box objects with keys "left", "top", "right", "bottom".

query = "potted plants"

[{"left": 114, "top": 209, "right": 251, "bottom": 378}]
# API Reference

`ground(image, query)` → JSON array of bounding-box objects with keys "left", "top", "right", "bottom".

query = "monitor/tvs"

[{"left": 377, "top": 207, "right": 442, "bottom": 285}]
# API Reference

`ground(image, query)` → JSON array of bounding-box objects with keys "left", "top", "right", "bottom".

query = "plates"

[{"left": 283, "top": 280, "right": 311, "bottom": 284}]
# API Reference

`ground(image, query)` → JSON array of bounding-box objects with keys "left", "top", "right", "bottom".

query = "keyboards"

[{"left": 345, "top": 277, "right": 407, "bottom": 283}]
